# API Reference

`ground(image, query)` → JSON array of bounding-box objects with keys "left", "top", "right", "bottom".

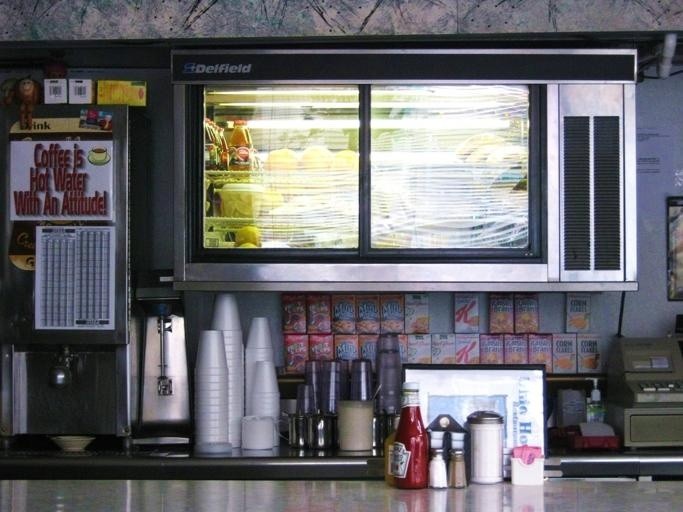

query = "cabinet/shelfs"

[
  {"left": 0, "top": 450, "right": 683, "bottom": 481},
  {"left": 166, "top": 49, "right": 637, "bottom": 294}
]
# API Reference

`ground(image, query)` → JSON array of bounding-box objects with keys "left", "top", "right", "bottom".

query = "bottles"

[
  {"left": 204, "top": 119, "right": 228, "bottom": 170},
  {"left": 383, "top": 382, "right": 505, "bottom": 491},
  {"left": 226, "top": 119, "right": 256, "bottom": 171}
]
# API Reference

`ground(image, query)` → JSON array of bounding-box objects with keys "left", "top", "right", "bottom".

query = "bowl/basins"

[{"left": 50, "top": 436, "right": 97, "bottom": 451}]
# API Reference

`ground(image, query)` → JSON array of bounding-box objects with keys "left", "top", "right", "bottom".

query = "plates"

[{"left": 87, "top": 154, "right": 111, "bottom": 166}]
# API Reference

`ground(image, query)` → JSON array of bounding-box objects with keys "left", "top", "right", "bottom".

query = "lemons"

[
  {"left": 233, "top": 225, "right": 261, "bottom": 248},
  {"left": 263, "top": 144, "right": 357, "bottom": 171}
]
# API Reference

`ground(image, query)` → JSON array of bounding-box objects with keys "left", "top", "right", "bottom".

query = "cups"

[
  {"left": 193, "top": 291, "right": 403, "bottom": 454},
  {"left": 88, "top": 146, "right": 107, "bottom": 161}
]
